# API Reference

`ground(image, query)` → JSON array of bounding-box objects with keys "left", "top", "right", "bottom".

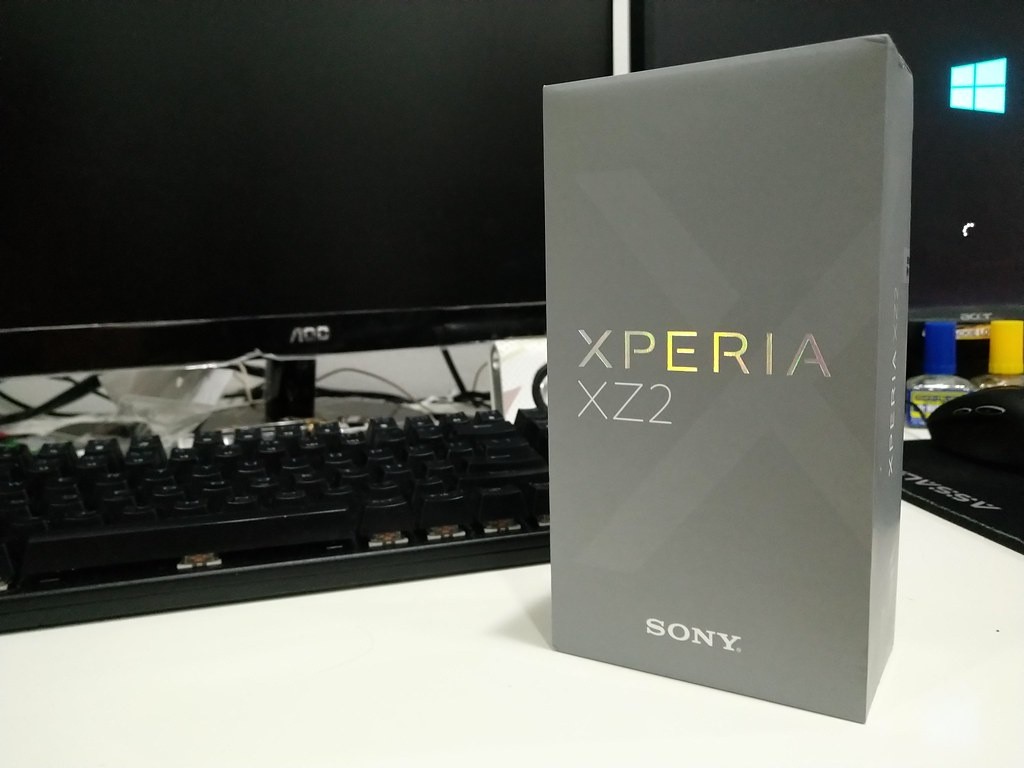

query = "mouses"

[{"left": 925, "top": 385, "right": 1024, "bottom": 462}]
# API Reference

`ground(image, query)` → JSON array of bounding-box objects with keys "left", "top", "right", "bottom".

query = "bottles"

[{"left": 906, "top": 376, "right": 1008, "bottom": 428}]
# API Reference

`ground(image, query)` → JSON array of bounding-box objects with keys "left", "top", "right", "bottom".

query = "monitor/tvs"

[
  {"left": 629, "top": 0, "right": 1024, "bottom": 381},
  {"left": 0, "top": 0, "right": 613, "bottom": 443}
]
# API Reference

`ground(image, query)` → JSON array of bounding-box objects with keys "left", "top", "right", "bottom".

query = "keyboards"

[{"left": 0, "top": 406, "right": 551, "bottom": 635}]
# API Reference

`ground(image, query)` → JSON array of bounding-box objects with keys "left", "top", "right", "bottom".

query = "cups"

[
  {"left": 923, "top": 320, "right": 956, "bottom": 376},
  {"left": 988, "top": 321, "right": 1024, "bottom": 376}
]
